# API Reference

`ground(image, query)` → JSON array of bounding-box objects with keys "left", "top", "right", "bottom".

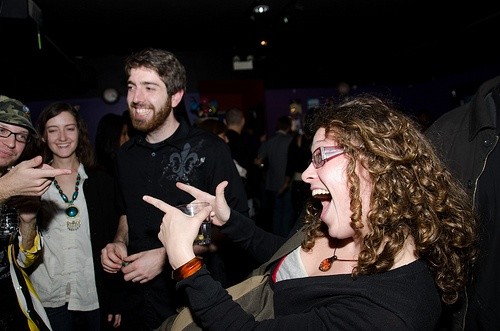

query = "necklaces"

[
  {"left": 318, "top": 239, "right": 359, "bottom": 272},
  {"left": 51, "top": 172, "right": 81, "bottom": 217}
]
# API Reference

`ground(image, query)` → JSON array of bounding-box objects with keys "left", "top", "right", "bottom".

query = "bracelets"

[{"left": 171, "top": 257, "right": 203, "bottom": 280}]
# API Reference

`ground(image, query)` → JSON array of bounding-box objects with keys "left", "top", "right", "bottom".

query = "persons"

[
  {"left": 95, "top": 47, "right": 248, "bottom": 331},
  {"left": 30, "top": 103, "right": 131, "bottom": 331},
  {"left": 0, "top": 95, "right": 71, "bottom": 331},
  {"left": 92, "top": 112, "right": 130, "bottom": 172},
  {"left": 218, "top": 105, "right": 331, "bottom": 234},
  {"left": 420, "top": 67, "right": 499, "bottom": 331},
  {"left": 141, "top": 98, "right": 477, "bottom": 331}
]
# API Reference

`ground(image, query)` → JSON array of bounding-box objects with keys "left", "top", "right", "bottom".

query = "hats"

[{"left": 0, "top": 95, "right": 38, "bottom": 138}]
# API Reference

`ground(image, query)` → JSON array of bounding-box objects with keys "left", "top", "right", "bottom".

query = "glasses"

[
  {"left": 311, "top": 146, "right": 352, "bottom": 169},
  {"left": 0, "top": 127, "right": 30, "bottom": 143}
]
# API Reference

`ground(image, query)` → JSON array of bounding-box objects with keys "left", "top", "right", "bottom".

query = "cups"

[{"left": 176, "top": 202, "right": 212, "bottom": 246}]
